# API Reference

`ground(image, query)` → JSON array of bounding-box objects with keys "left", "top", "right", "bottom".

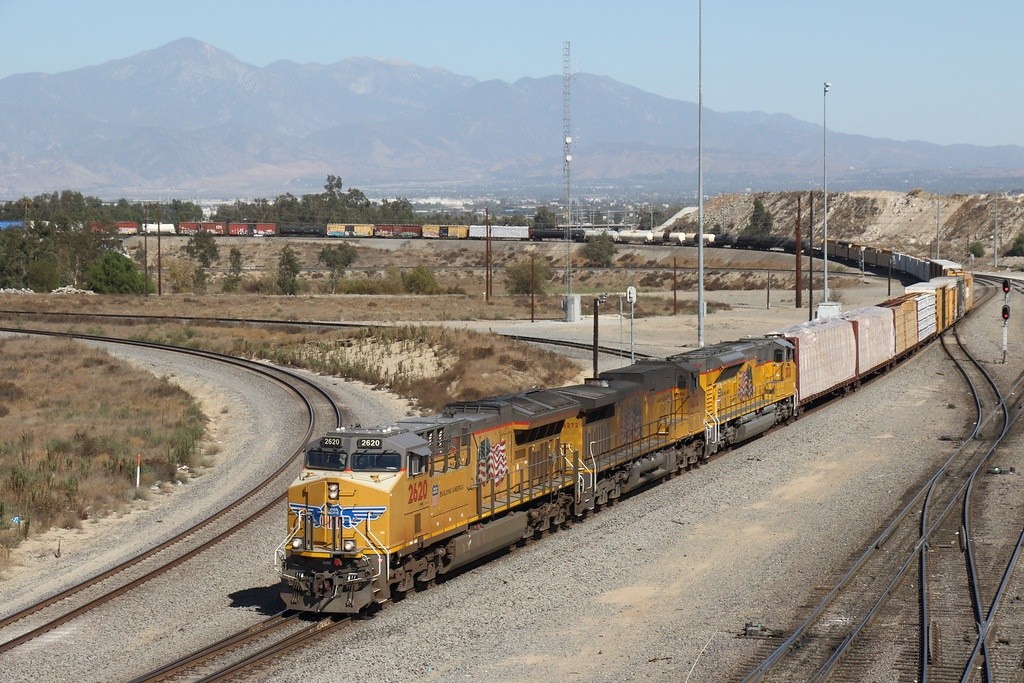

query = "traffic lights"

[
  {"left": 1002, "top": 305, "right": 1010, "bottom": 319},
  {"left": 1003, "top": 279, "right": 1010, "bottom": 293}
]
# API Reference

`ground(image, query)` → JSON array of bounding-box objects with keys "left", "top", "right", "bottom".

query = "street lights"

[
  {"left": 566, "top": 136, "right": 572, "bottom": 293},
  {"left": 823, "top": 81, "right": 832, "bottom": 303}
]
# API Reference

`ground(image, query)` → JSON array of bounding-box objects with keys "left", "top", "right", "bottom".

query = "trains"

[
  {"left": 1, "top": 221, "right": 813, "bottom": 256},
  {"left": 276, "top": 236, "right": 973, "bottom": 624}
]
